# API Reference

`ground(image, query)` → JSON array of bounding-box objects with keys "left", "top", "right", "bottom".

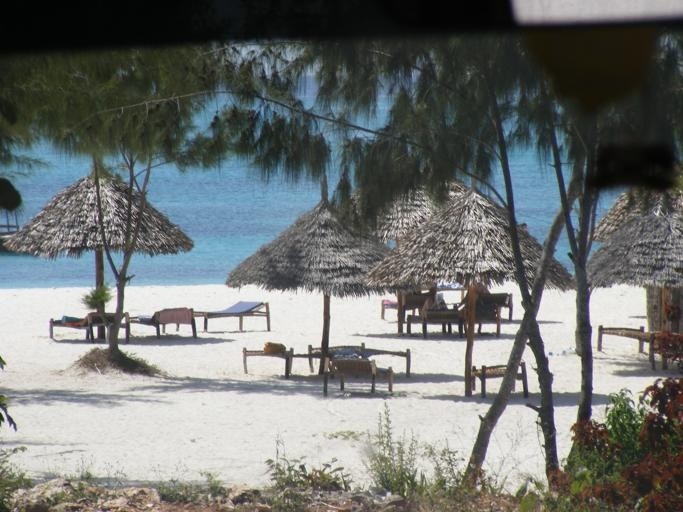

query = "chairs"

[
  {"left": 597, "top": 325, "right": 682, "bottom": 370},
  {"left": 472, "top": 361, "right": 528, "bottom": 398},
  {"left": 381, "top": 290, "right": 512, "bottom": 337},
  {"left": 243, "top": 343, "right": 410, "bottom": 395},
  {"left": 50, "top": 300, "right": 270, "bottom": 342}
]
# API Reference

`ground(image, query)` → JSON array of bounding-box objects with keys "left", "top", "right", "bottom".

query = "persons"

[
  {"left": 422, "top": 286, "right": 452, "bottom": 336},
  {"left": 454, "top": 281, "right": 491, "bottom": 337}
]
